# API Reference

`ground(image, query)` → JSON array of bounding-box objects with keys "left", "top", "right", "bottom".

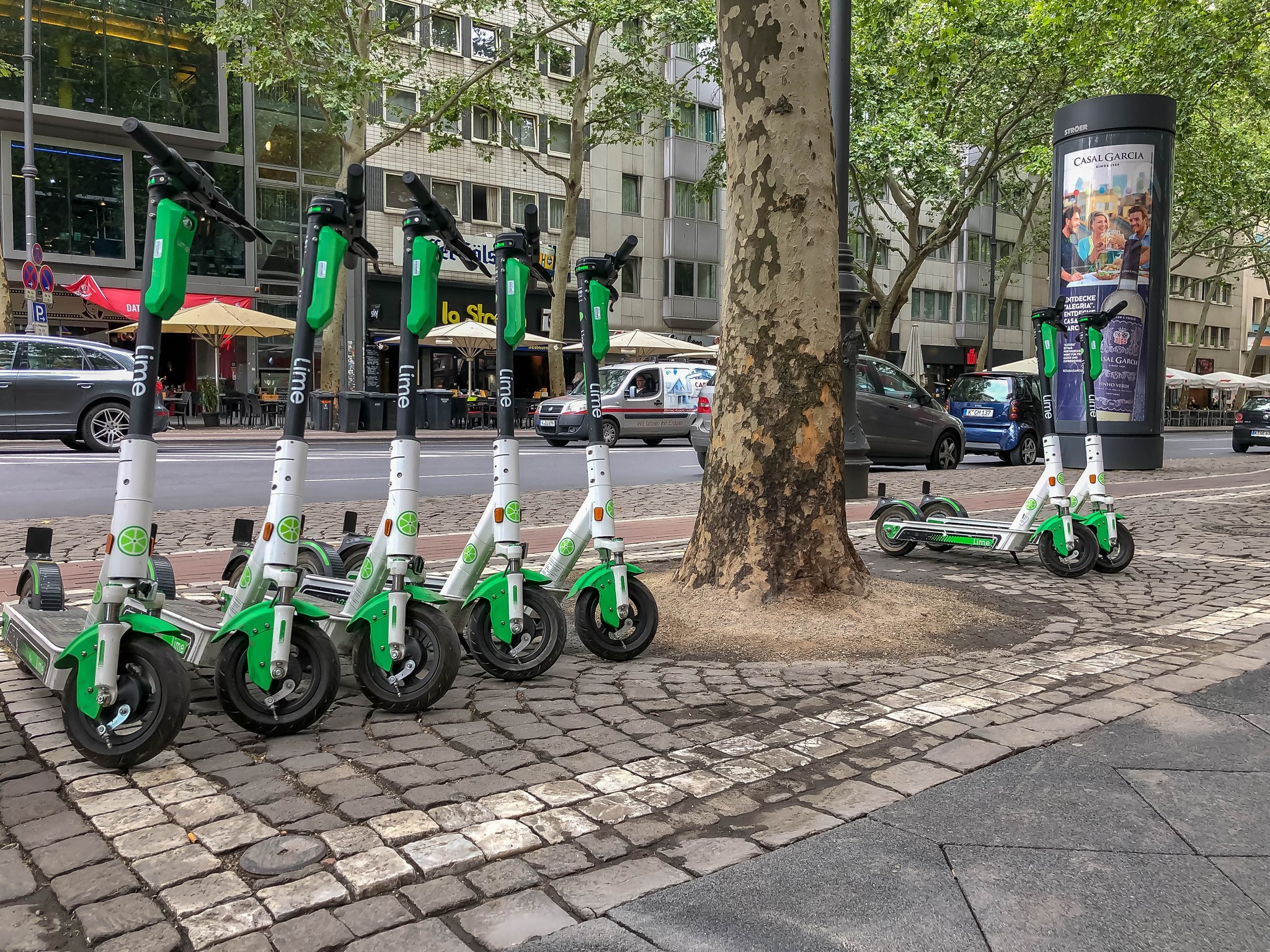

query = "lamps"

[{"left": 474, "top": 231, "right": 493, "bottom": 238}]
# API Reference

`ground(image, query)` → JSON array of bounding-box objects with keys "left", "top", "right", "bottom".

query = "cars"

[{"left": 1232, "top": 394, "right": 1270, "bottom": 453}]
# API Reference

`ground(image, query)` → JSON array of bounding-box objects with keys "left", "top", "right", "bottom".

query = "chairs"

[
  {"left": 1164, "top": 408, "right": 1236, "bottom": 427},
  {"left": 451, "top": 397, "right": 541, "bottom": 429},
  {"left": 165, "top": 392, "right": 191, "bottom": 430},
  {"left": 224, "top": 390, "right": 284, "bottom": 431}
]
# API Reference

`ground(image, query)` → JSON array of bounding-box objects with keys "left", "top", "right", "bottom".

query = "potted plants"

[{"left": 198, "top": 375, "right": 221, "bottom": 427}]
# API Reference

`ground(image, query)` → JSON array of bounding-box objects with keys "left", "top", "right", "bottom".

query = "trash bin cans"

[
  {"left": 424, "top": 388, "right": 454, "bottom": 430},
  {"left": 333, "top": 391, "right": 365, "bottom": 433},
  {"left": 382, "top": 393, "right": 398, "bottom": 430},
  {"left": 416, "top": 389, "right": 427, "bottom": 429},
  {"left": 351, "top": 391, "right": 387, "bottom": 431},
  {"left": 307, "top": 391, "right": 336, "bottom": 431}
]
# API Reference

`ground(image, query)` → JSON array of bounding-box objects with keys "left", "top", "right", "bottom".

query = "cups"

[
  {"left": 1100, "top": 229, "right": 1124, "bottom": 251},
  {"left": 1095, "top": 259, "right": 1101, "bottom": 271}
]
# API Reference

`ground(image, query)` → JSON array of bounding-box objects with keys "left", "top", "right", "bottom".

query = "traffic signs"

[{"left": 32, "top": 302, "right": 46, "bottom": 325}]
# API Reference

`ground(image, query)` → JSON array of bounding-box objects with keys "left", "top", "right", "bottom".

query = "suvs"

[
  {"left": 0, "top": 333, "right": 170, "bottom": 453},
  {"left": 944, "top": 370, "right": 1044, "bottom": 466},
  {"left": 533, "top": 356, "right": 717, "bottom": 448},
  {"left": 690, "top": 351, "right": 967, "bottom": 471}
]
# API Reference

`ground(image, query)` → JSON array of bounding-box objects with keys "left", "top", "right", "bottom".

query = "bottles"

[{"left": 1094, "top": 239, "right": 1145, "bottom": 422}]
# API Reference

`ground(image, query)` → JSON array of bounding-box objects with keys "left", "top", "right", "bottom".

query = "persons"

[
  {"left": 1178, "top": 394, "right": 1251, "bottom": 426},
  {"left": 572, "top": 372, "right": 584, "bottom": 392},
  {"left": 1061, "top": 205, "right": 1150, "bottom": 288},
  {"left": 1198, "top": 359, "right": 1213, "bottom": 373},
  {"left": 636, "top": 374, "right": 657, "bottom": 398}
]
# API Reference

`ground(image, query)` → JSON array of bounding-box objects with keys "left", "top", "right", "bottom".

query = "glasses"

[{"left": 636, "top": 380, "right": 644, "bottom": 382}]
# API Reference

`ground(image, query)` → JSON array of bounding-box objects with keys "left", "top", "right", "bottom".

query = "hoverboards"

[
  {"left": 919, "top": 300, "right": 1135, "bottom": 575},
  {"left": 869, "top": 294, "right": 1101, "bottom": 581},
  {"left": 0, "top": 115, "right": 660, "bottom": 769}
]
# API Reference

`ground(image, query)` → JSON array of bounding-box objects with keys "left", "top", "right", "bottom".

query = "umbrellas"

[
  {"left": 1165, "top": 367, "right": 1270, "bottom": 410},
  {"left": 562, "top": 328, "right": 718, "bottom": 362},
  {"left": 105, "top": 298, "right": 297, "bottom": 413},
  {"left": 983, "top": 356, "right": 1039, "bottom": 375},
  {"left": 902, "top": 324, "right": 926, "bottom": 394},
  {"left": 667, "top": 344, "right": 720, "bottom": 359},
  {"left": 374, "top": 318, "right": 567, "bottom": 397}
]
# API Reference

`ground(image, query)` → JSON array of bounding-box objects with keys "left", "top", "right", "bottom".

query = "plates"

[{"left": 1092, "top": 272, "right": 1120, "bottom": 280}]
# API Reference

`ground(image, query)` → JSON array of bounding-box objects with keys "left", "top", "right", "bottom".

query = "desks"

[
  {"left": 466, "top": 400, "right": 497, "bottom": 429},
  {"left": 223, "top": 397, "right": 251, "bottom": 428},
  {"left": 257, "top": 399, "right": 288, "bottom": 430},
  {"left": 174, "top": 391, "right": 192, "bottom": 417},
  {"left": 163, "top": 397, "right": 185, "bottom": 429}
]
